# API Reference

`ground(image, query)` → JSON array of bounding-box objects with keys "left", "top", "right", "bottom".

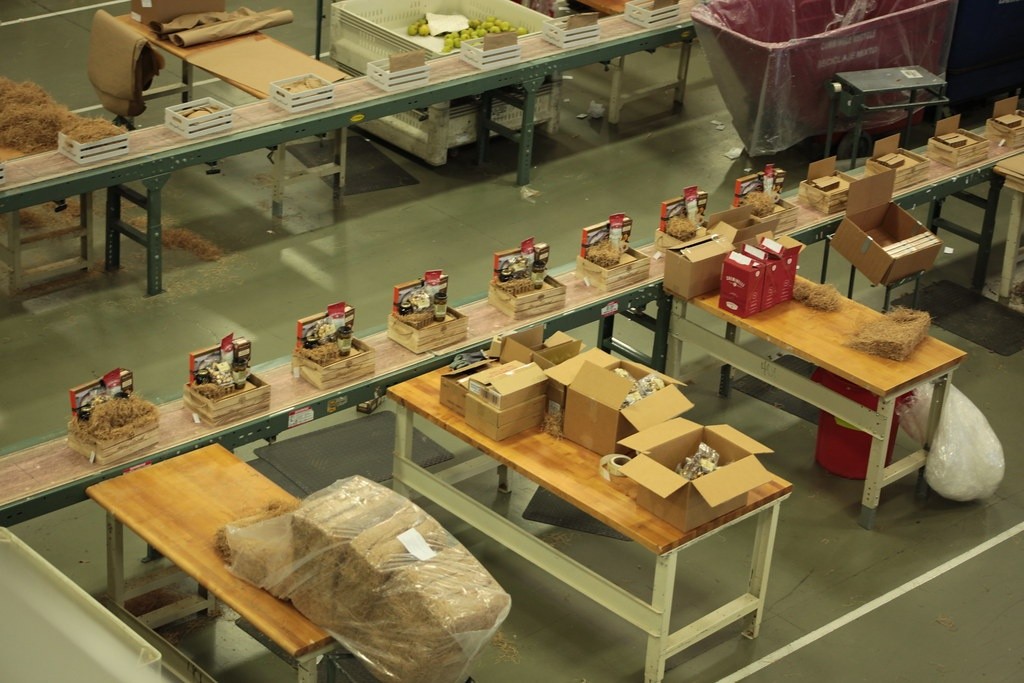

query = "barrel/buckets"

[{"left": 812, "top": 365, "right": 917, "bottom": 480}]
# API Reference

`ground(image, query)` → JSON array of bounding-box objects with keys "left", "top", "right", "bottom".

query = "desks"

[
  {"left": 994, "top": 152, "right": 1024, "bottom": 315},
  {"left": 0, "top": 144, "right": 96, "bottom": 293},
  {"left": 663, "top": 275, "right": 969, "bottom": 528},
  {"left": 387, "top": 349, "right": 794, "bottom": 683},
  {"left": 84, "top": 442, "right": 341, "bottom": 683},
  {"left": 115, "top": 13, "right": 355, "bottom": 218}
]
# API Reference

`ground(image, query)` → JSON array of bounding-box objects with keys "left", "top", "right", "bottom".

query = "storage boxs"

[
  {"left": 164, "top": 96, "right": 234, "bottom": 140},
  {"left": 625, "top": 0, "right": 680, "bottom": 28},
  {"left": 57, "top": 121, "right": 128, "bottom": 165},
  {"left": 760, "top": 234, "right": 807, "bottom": 305},
  {"left": 719, "top": 251, "right": 765, "bottom": 317},
  {"left": 830, "top": 168, "right": 944, "bottom": 286},
  {"left": 654, "top": 224, "right": 706, "bottom": 253},
  {"left": 987, "top": 110, "right": 1024, "bottom": 149},
  {"left": 367, "top": 58, "right": 430, "bottom": 92},
  {"left": 731, "top": 197, "right": 799, "bottom": 235},
  {"left": 330, "top": 0, "right": 564, "bottom": 167},
  {"left": 488, "top": 272, "right": 567, "bottom": 321},
  {"left": 928, "top": 128, "right": 990, "bottom": 169},
  {"left": 741, "top": 243, "right": 782, "bottom": 312},
  {"left": 864, "top": 148, "right": 930, "bottom": 191},
  {"left": 271, "top": 73, "right": 334, "bottom": 113},
  {"left": 439, "top": 321, "right": 774, "bottom": 533},
  {"left": 542, "top": 16, "right": 600, "bottom": 49},
  {"left": 387, "top": 306, "right": 469, "bottom": 354},
  {"left": 708, "top": 205, "right": 779, "bottom": 250},
  {"left": 291, "top": 336, "right": 377, "bottom": 390},
  {"left": 68, "top": 418, "right": 162, "bottom": 465},
  {"left": 664, "top": 234, "right": 736, "bottom": 299},
  {"left": 576, "top": 245, "right": 651, "bottom": 292},
  {"left": 460, "top": 37, "right": 521, "bottom": 71},
  {"left": 800, "top": 169, "right": 860, "bottom": 214},
  {"left": 182, "top": 369, "right": 271, "bottom": 428}
]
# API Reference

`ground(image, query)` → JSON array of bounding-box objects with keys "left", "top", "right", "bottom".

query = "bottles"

[
  {"left": 500, "top": 268, "right": 513, "bottom": 282},
  {"left": 304, "top": 333, "right": 321, "bottom": 350},
  {"left": 336, "top": 324, "right": 354, "bottom": 358},
  {"left": 433, "top": 291, "right": 448, "bottom": 323},
  {"left": 531, "top": 259, "right": 545, "bottom": 290},
  {"left": 77, "top": 404, "right": 93, "bottom": 422},
  {"left": 115, "top": 390, "right": 129, "bottom": 401},
  {"left": 230, "top": 355, "right": 249, "bottom": 391},
  {"left": 194, "top": 369, "right": 216, "bottom": 385}
]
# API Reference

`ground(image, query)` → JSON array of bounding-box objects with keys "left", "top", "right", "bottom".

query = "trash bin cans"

[
  {"left": 689, "top": 0, "right": 958, "bottom": 156},
  {"left": 808, "top": 364, "right": 916, "bottom": 481}
]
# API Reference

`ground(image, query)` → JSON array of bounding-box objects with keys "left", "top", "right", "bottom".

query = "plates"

[{"left": 398, "top": 300, "right": 413, "bottom": 316}]
